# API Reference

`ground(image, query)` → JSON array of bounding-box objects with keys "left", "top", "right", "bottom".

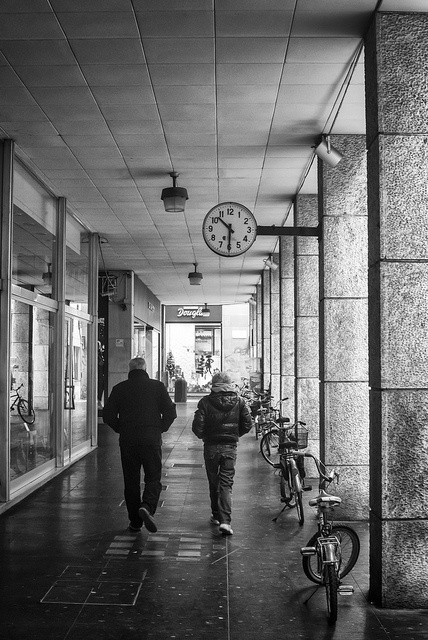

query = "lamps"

[
  {"left": 201, "top": 303, "right": 210, "bottom": 316},
  {"left": 187, "top": 263, "right": 203, "bottom": 285},
  {"left": 160, "top": 172, "right": 189, "bottom": 213}
]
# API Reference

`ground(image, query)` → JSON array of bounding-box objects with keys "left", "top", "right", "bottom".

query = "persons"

[
  {"left": 165, "top": 351, "right": 176, "bottom": 379},
  {"left": 103, "top": 356, "right": 177, "bottom": 534},
  {"left": 191, "top": 372, "right": 253, "bottom": 535}
]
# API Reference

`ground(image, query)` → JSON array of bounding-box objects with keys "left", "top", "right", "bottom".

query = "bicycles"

[
  {"left": 247, "top": 396, "right": 274, "bottom": 459},
  {"left": 291, "top": 451, "right": 360, "bottom": 622},
  {"left": 10, "top": 384, "right": 35, "bottom": 423},
  {"left": 260, "top": 421, "right": 308, "bottom": 525},
  {"left": 264, "top": 397, "right": 289, "bottom": 467}
]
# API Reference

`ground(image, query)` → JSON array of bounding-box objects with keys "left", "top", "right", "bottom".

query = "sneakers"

[
  {"left": 138, "top": 507, "right": 157, "bottom": 533},
  {"left": 218, "top": 523, "right": 234, "bottom": 535},
  {"left": 210, "top": 513, "right": 220, "bottom": 525},
  {"left": 130, "top": 522, "right": 141, "bottom": 531}
]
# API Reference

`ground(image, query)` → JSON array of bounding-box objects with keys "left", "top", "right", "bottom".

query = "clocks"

[{"left": 200, "top": 203, "right": 258, "bottom": 256}]
